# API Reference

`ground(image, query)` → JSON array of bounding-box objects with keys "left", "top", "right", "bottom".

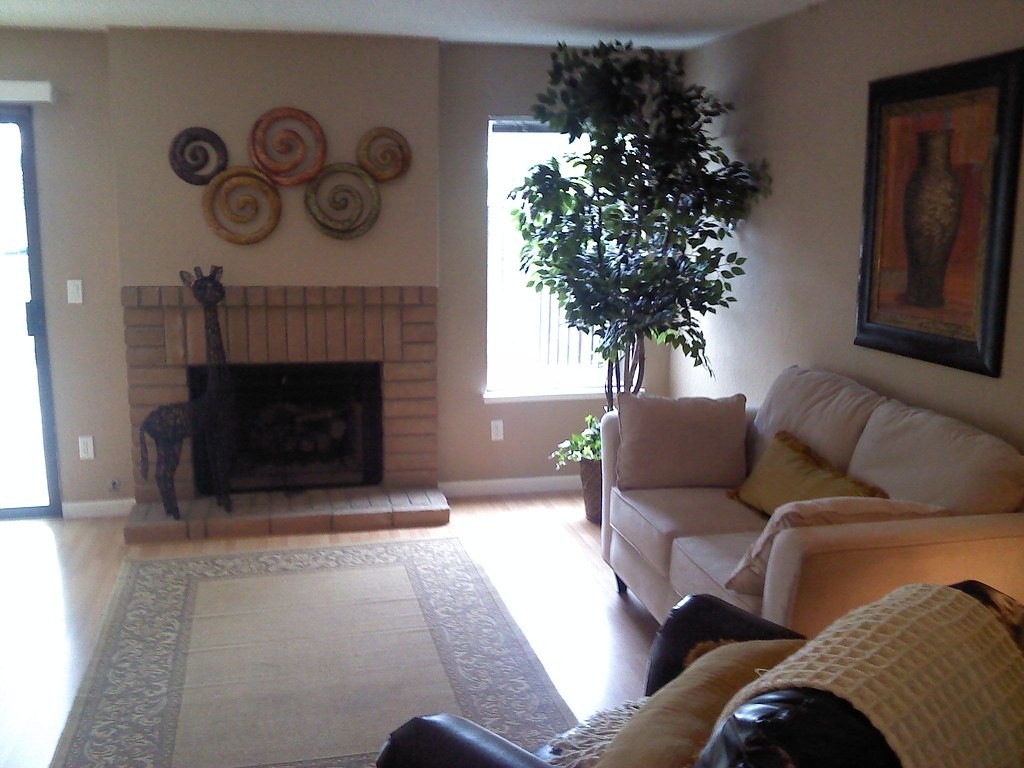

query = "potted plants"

[{"left": 506, "top": 39, "right": 774, "bottom": 526}]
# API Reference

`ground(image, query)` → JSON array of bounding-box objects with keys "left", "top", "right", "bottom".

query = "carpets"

[{"left": 50, "top": 535, "right": 580, "bottom": 768}]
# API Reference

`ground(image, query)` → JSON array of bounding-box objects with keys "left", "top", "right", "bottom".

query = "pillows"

[
  {"left": 616, "top": 386, "right": 749, "bottom": 491},
  {"left": 601, "top": 637, "right": 807, "bottom": 762},
  {"left": 723, "top": 430, "right": 949, "bottom": 591}
]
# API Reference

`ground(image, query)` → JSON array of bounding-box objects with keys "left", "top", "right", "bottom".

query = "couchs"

[
  {"left": 601, "top": 364, "right": 1024, "bottom": 659},
  {"left": 375, "top": 580, "right": 1024, "bottom": 768}
]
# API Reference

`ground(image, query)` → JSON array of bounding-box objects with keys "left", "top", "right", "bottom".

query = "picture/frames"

[{"left": 854, "top": 49, "right": 1024, "bottom": 382}]
